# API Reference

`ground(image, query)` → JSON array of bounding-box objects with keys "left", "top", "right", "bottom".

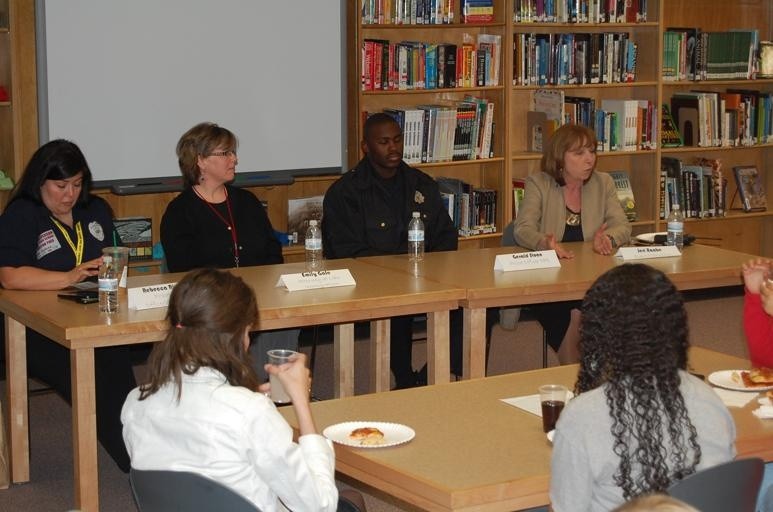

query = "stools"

[
  {"left": 26, "top": 367, "right": 55, "bottom": 395},
  {"left": 341, "top": 314, "right": 461, "bottom": 386}
]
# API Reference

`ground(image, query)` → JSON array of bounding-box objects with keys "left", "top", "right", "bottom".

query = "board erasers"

[{"left": 117, "top": 182, "right": 134, "bottom": 189}]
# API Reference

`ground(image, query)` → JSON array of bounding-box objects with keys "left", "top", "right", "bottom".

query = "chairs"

[
  {"left": 609, "top": 493, "right": 702, "bottom": 512},
  {"left": 486, "top": 218, "right": 580, "bottom": 379},
  {"left": 124, "top": 459, "right": 263, "bottom": 512},
  {"left": 647, "top": 454, "right": 766, "bottom": 511}
]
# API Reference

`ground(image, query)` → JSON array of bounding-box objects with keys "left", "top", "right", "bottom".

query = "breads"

[
  {"left": 349, "top": 427, "right": 386, "bottom": 446},
  {"left": 741, "top": 364, "right": 773, "bottom": 388}
]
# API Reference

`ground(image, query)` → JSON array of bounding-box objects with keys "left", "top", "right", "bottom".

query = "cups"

[
  {"left": 537, "top": 383, "right": 567, "bottom": 434},
  {"left": 266, "top": 350, "right": 301, "bottom": 405}
]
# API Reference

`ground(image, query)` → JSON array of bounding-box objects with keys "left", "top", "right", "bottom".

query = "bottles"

[
  {"left": 407, "top": 211, "right": 426, "bottom": 263},
  {"left": 304, "top": 219, "right": 323, "bottom": 271},
  {"left": 409, "top": 263, "right": 425, "bottom": 291},
  {"left": 667, "top": 204, "right": 684, "bottom": 254},
  {"left": 97, "top": 257, "right": 119, "bottom": 314}
]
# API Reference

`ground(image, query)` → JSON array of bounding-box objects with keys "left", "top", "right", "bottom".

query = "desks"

[
  {"left": 270, "top": 341, "right": 772, "bottom": 512},
  {"left": 353, "top": 239, "right": 773, "bottom": 399},
  {"left": 0, "top": 256, "right": 469, "bottom": 512}
]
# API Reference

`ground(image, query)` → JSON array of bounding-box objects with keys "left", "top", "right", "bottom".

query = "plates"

[
  {"left": 706, "top": 369, "right": 772, "bottom": 392},
  {"left": 323, "top": 419, "right": 417, "bottom": 449}
]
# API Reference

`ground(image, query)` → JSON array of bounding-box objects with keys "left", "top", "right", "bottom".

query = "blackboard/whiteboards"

[{"left": 34, "top": 0, "right": 349, "bottom": 196}]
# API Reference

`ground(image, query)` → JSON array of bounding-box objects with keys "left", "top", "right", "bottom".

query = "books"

[
  {"left": 662, "top": 87, "right": 773, "bottom": 146},
  {"left": 359, "top": 0, "right": 496, "bottom": 26},
  {"left": 436, "top": 174, "right": 498, "bottom": 237},
  {"left": 513, "top": 170, "right": 638, "bottom": 221},
  {"left": 660, "top": 27, "right": 772, "bottom": 82},
  {"left": 514, "top": 31, "right": 639, "bottom": 86},
  {"left": 527, "top": 89, "right": 659, "bottom": 151},
  {"left": 362, "top": 94, "right": 496, "bottom": 166},
  {"left": 361, "top": 33, "right": 502, "bottom": 92},
  {"left": 514, "top": 0, "right": 657, "bottom": 24},
  {"left": 660, "top": 156, "right": 768, "bottom": 220}
]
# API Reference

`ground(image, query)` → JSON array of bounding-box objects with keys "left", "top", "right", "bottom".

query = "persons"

[
  {"left": 0, "top": 138, "right": 139, "bottom": 474},
  {"left": 160, "top": 121, "right": 304, "bottom": 388},
  {"left": 320, "top": 113, "right": 489, "bottom": 386},
  {"left": 740, "top": 257, "right": 773, "bottom": 512},
  {"left": 549, "top": 262, "right": 737, "bottom": 512},
  {"left": 119, "top": 270, "right": 339, "bottom": 512},
  {"left": 513, "top": 124, "right": 633, "bottom": 366}
]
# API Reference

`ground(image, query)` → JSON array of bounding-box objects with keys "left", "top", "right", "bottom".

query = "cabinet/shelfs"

[
  {"left": 0, "top": 23, "right": 15, "bottom": 194},
  {"left": 340, "top": 1, "right": 772, "bottom": 266}
]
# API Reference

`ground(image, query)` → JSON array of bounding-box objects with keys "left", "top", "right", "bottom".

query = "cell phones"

[{"left": 57, "top": 291, "right": 105, "bottom": 304}]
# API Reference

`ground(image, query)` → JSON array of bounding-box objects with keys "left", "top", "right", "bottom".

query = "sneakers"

[{"left": 393, "top": 360, "right": 433, "bottom": 392}]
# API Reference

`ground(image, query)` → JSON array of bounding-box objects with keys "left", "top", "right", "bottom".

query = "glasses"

[{"left": 200, "top": 149, "right": 238, "bottom": 158}]
